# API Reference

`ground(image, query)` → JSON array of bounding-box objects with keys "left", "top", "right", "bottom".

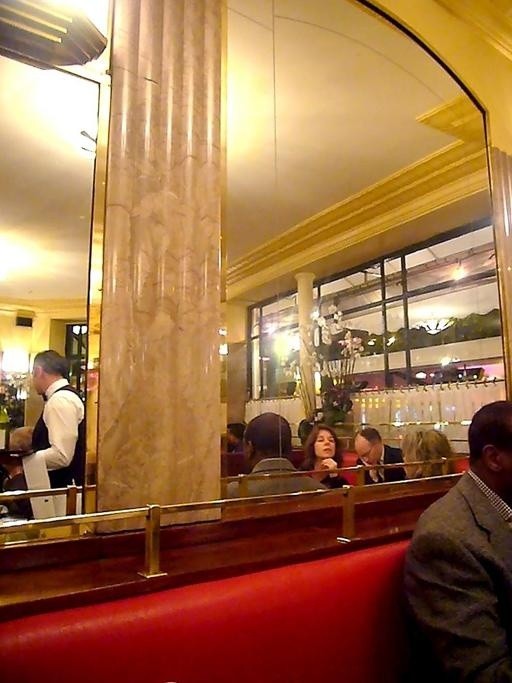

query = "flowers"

[{"left": 282, "top": 302, "right": 370, "bottom": 425}]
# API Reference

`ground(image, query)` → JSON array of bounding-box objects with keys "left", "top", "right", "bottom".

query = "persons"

[
  {"left": 4, "top": 424, "right": 34, "bottom": 519},
  {"left": 20, "top": 348, "right": 87, "bottom": 518},
  {"left": 399, "top": 397, "right": 511, "bottom": 682},
  {"left": 220, "top": 409, "right": 455, "bottom": 500}
]
